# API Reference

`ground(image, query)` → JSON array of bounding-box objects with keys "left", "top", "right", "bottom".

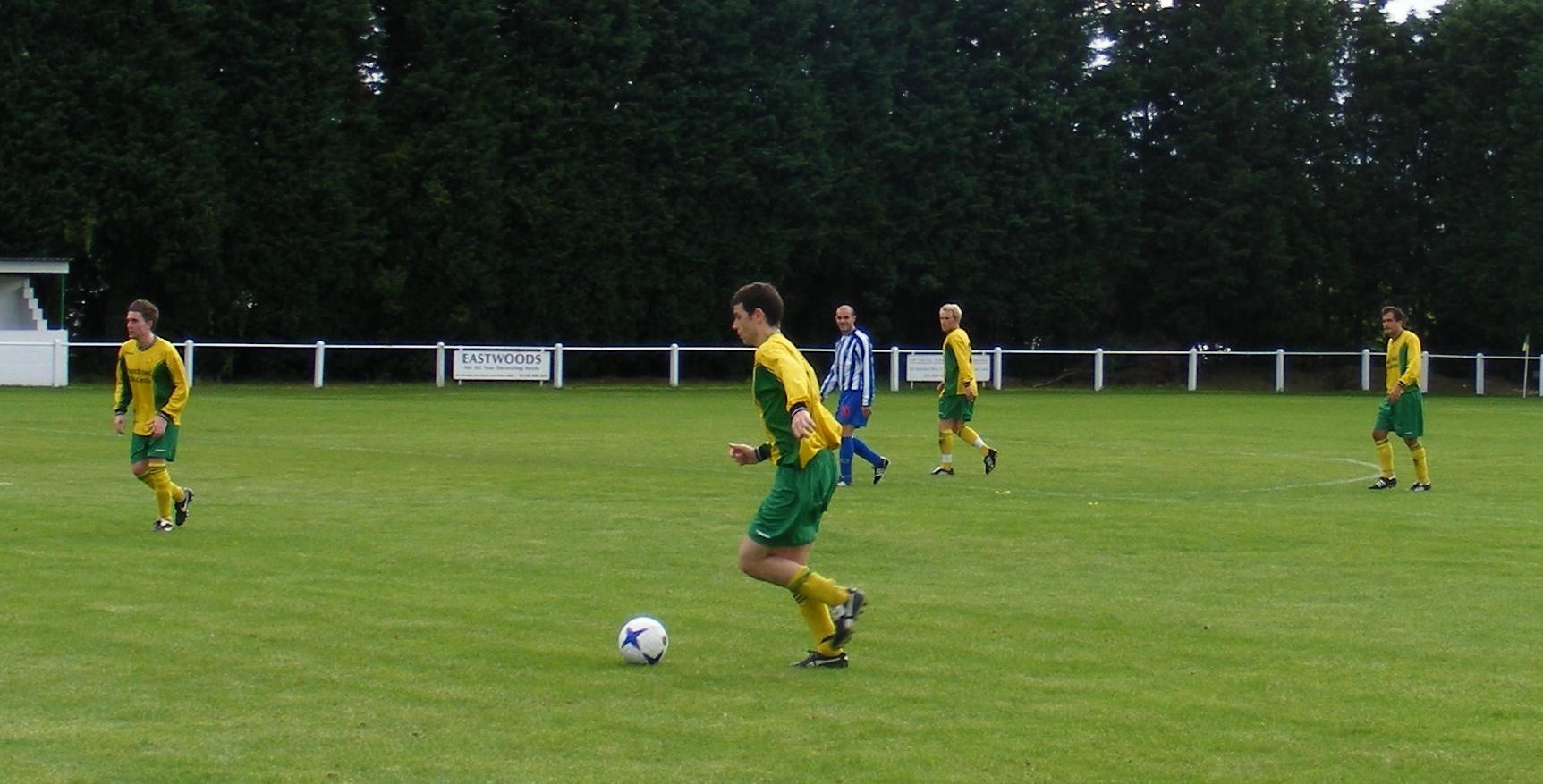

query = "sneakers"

[
  {"left": 176, "top": 487, "right": 194, "bottom": 525},
  {"left": 835, "top": 477, "right": 852, "bottom": 487},
  {"left": 150, "top": 518, "right": 173, "bottom": 533},
  {"left": 984, "top": 448, "right": 999, "bottom": 475},
  {"left": 929, "top": 466, "right": 954, "bottom": 476},
  {"left": 1404, "top": 479, "right": 1431, "bottom": 492},
  {"left": 1368, "top": 475, "right": 1397, "bottom": 490},
  {"left": 789, "top": 650, "right": 849, "bottom": 669},
  {"left": 872, "top": 455, "right": 890, "bottom": 485},
  {"left": 832, "top": 589, "right": 866, "bottom": 648}
]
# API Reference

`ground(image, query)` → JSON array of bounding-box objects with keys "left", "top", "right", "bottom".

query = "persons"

[
  {"left": 821, "top": 304, "right": 891, "bottom": 486},
  {"left": 729, "top": 281, "right": 868, "bottom": 671},
  {"left": 1367, "top": 306, "right": 1432, "bottom": 491},
  {"left": 930, "top": 304, "right": 998, "bottom": 476},
  {"left": 113, "top": 298, "right": 195, "bottom": 532}
]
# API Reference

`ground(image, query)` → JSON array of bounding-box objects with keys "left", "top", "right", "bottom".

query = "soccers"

[{"left": 618, "top": 617, "right": 668, "bottom": 666}]
